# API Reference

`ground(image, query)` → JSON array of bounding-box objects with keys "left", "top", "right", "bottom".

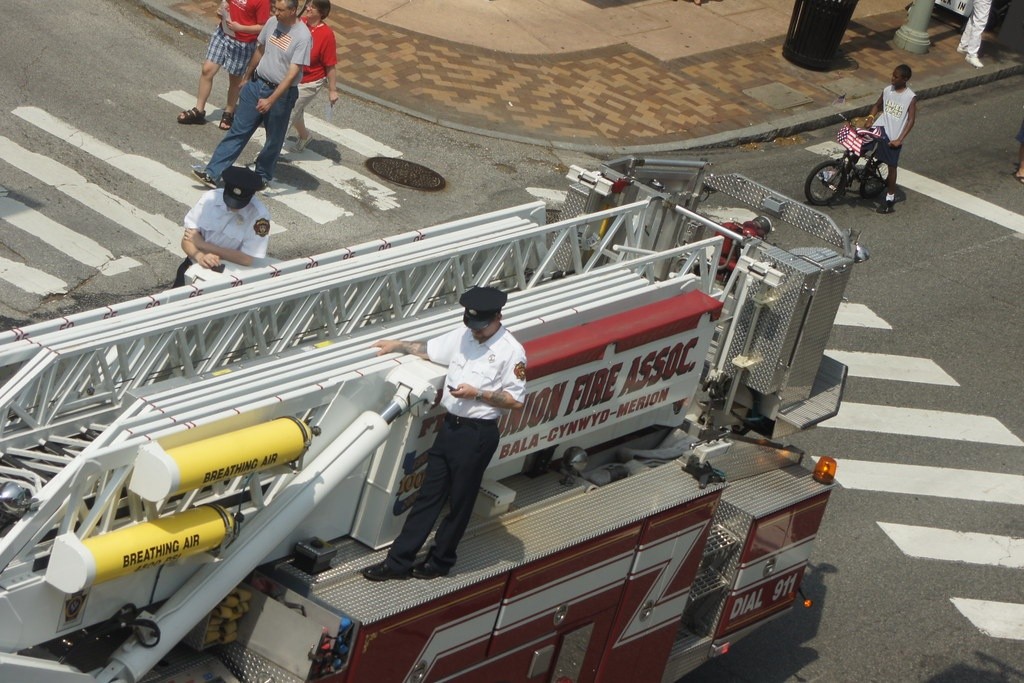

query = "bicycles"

[{"left": 804, "top": 114, "right": 902, "bottom": 205}]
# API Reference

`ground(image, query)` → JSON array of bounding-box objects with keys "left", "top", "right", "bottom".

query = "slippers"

[{"left": 1014, "top": 171, "right": 1024, "bottom": 184}]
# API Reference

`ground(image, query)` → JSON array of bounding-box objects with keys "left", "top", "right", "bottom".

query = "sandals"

[
  {"left": 219, "top": 111, "right": 234, "bottom": 130},
  {"left": 178, "top": 107, "right": 205, "bottom": 124}
]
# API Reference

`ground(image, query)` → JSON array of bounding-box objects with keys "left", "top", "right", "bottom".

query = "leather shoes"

[
  {"left": 363, "top": 559, "right": 410, "bottom": 581},
  {"left": 410, "top": 561, "right": 449, "bottom": 579}
]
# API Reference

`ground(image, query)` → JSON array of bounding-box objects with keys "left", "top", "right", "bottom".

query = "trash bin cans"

[{"left": 782, "top": 0, "right": 863, "bottom": 70}]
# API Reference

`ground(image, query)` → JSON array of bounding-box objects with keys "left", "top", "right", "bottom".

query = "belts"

[{"left": 258, "top": 77, "right": 278, "bottom": 89}]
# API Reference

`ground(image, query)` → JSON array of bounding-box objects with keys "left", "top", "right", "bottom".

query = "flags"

[{"left": 856, "top": 126, "right": 882, "bottom": 144}]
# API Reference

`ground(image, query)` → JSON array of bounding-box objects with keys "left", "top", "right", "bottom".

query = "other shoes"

[
  {"left": 291, "top": 135, "right": 312, "bottom": 153},
  {"left": 193, "top": 169, "right": 218, "bottom": 189},
  {"left": 876, "top": 197, "right": 896, "bottom": 213},
  {"left": 253, "top": 155, "right": 259, "bottom": 162},
  {"left": 262, "top": 180, "right": 267, "bottom": 190}
]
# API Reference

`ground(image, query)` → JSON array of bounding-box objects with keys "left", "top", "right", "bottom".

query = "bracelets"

[{"left": 868, "top": 115, "right": 874, "bottom": 119}]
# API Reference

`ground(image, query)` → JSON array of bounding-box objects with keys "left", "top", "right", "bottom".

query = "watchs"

[{"left": 474, "top": 391, "right": 483, "bottom": 402}]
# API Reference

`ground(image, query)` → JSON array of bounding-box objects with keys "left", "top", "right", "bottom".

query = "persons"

[
  {"left": 363, "top": 286, "right": 528, "bottom": 581},
  {"left": 957, "top": 0, "right": 992, "bottom": 68},
  {"left": 176, "top": 0, "right": 338, "bottom": 189},
  {"left": 846, "top": 64, "right": 917, "bottom": 208},
  {"left": 171, "top": 167, "right": 271, "bottom": 289},
  {"left": 1012, "top": 119, "right": 1024, "bottom": 184}
]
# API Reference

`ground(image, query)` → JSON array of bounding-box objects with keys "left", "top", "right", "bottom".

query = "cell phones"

[
  {"left": 448, "top": 385, "right": 456, "bottom": 392},
  {"left": 212, "top": 264, "right": 225, "bottom": 273}
]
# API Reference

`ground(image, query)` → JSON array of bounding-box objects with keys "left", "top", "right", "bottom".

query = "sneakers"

[
  {"left": 965, "top": 54, "right": 984, "bottom": 68},
  {"left": 956, "top": 46, "right": 968, "bottom": 55}
]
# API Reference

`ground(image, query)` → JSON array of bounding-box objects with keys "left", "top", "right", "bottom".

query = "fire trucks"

[{"left": 0, "top": 159, "right": 869, "bottom": 683}]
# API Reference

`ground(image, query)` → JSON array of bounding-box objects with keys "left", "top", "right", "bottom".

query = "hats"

[
  {"left": 222, "top": 165, "right": 263, "bottom": 209},
  {"left": 459, "top": 286, "right": 508, "bottom": 329}
]
